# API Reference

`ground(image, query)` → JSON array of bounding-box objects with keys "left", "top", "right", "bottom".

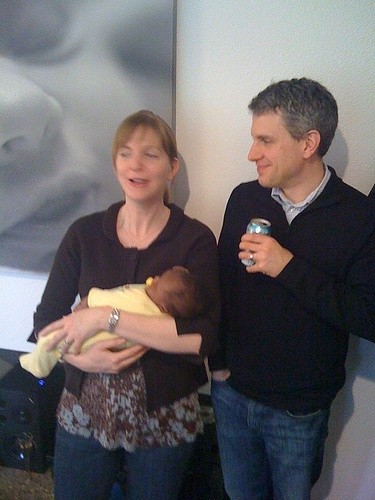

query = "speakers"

[{"left": 0, "top": 362, "right": 66, "bottom": 473}]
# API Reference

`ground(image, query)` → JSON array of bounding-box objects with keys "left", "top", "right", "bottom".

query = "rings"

[
  {"left": 249, "top": 252, "right": 255, "bottom": 261},
  {"left": 64, "top": 336, "right": 73, "bottom": 346}
]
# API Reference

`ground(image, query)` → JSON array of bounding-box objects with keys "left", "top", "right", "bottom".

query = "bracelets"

[{"left": 107, "top": 307, "right": 121, "bottom": 333}]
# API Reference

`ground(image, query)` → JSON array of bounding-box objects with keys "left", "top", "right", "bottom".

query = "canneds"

[{"left": 241, "top": 218, "right": 271, "bottom": 266}]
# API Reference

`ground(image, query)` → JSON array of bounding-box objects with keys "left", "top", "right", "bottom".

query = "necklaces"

[{"left": 119, "top": 207, "right": 169, "bottom": 248}]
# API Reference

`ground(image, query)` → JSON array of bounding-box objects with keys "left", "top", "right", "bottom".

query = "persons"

[
  {"left": 18, "top": 266, "right": 206, "bottom": 379},
  {"left": 33, "top": 110, "right": 221, "bottom": 500},
  {"left": 207, "top": 76, "right": 375, "bottom": 500},
  {"left": 0, "top": 0, "right": 142, "bottom": 276}
]
